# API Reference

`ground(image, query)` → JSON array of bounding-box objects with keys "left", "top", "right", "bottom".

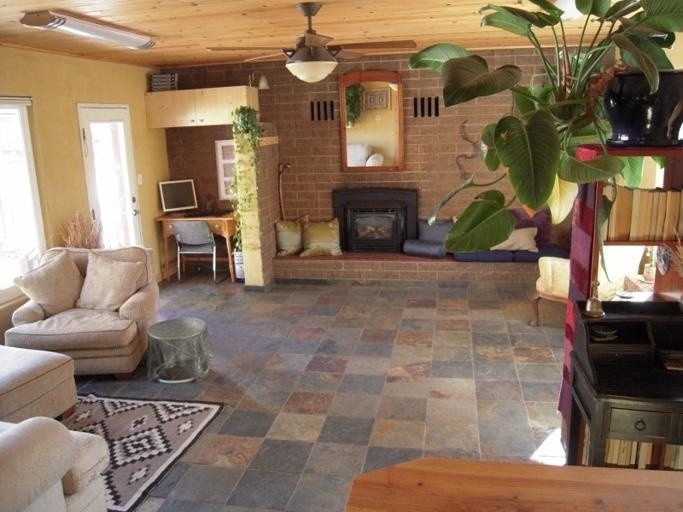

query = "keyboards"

[{"left": 185, "top": 209, "right": 224, "bottom": 217}]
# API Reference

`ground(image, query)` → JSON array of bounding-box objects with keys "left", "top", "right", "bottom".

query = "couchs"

[
  {"left": 0, "top": 417, "right": 112, "bottom": 511},
  {"left": 3, "top": 243, "right": 160, "bottom": 382},
  {"left": 0, "top": 343, "right": 79, "bottom": 425}
]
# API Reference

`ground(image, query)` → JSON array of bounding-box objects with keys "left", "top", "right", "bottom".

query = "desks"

[
  {"left": 339, "top": 453, "right": 681, "bottom": 510},
  {"left": 153, "top": 212, "right": 239, "bottom": 287}
]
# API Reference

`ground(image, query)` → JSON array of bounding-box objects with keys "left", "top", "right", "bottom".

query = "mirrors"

[{"left": 337, "top": 68, "right": 404, "bottom": 174}]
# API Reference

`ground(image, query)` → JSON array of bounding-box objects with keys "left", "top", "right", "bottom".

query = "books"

[
  {"left": 580, "top": 419, "right": 683, "bottom": 471},
  {"left": 605, "top": 182, "right": 681, "bottom": 242}
]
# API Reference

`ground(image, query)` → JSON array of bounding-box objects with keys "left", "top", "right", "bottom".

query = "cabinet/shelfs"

[
  {"left": 141, "top": 82, "right": 263, "bottom": 130},
  {"left": 557, "top": 297, "right": 683, "bottom": 474},
  {"left": 558, "top": 145, "right": 683, "bottom": 458}
]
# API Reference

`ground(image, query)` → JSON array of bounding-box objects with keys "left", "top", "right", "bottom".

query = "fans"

[{"left": 202, "top": 2, "right": 418, "bottom": 86}]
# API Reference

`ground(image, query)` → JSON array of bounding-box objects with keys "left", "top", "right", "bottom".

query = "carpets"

[{"left": 55, "top": 392, "right": 227, "bottom": 511}]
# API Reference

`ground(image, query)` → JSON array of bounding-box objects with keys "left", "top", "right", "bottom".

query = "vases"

[
  {"left": 604, "top": 67, "right": 683, "bottom": 147},
  {"left": 232, "top": 248, "right": 248, "bottom": 283}
]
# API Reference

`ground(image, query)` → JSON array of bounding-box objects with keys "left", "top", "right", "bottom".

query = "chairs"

[
  {"left": 170, "top": 219, "right": 230, "bottom": 283},
  {"left": 526, "top": 251, "right": 575, "bottom": 327}
]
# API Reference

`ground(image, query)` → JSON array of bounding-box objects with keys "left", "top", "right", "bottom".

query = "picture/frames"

[{"left": 361, "top": 87, "right": 394, "bottom": 114}]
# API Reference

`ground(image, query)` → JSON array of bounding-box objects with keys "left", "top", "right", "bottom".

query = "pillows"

[
  {"left": 9, "top": 248, "right": 84, "bottom": 318},
  {"left": 508, "top": 207, "right": 551, "bottom": 247},
  {"left": 417, "top": 219, "right": 453, "bottom": 246},
  {"left": 402, "top": 238, "right": 449, "bottom": 256},
  {"left": 488, "top": 225, "right": 540, "bottom": 253},
  {"left": 73, "top": 251, "right": 146, "bottom": 315},
  {"left": 298, "top": 217, "right": 344, "bottom": 257},
  {"left": 274, "top": 214, "right": 311, "bottom": 260}
]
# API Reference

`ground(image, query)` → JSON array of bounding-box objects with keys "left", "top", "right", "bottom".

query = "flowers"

[{"left": 229, "top": 198, "right": 244, "bottom": 251}]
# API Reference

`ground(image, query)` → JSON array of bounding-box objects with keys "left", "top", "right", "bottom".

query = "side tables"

[{"left": 144, "top": 317, "right": 213, "bottom": 385}]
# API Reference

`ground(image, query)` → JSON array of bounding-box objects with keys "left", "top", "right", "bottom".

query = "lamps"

[
  {"left": 285, "top": 46, "right": 341, "bottom": 86},
  {"left": 18, "top": 8, "right": 157, "bottom": 56},
  {"left": 247, "top": 68, "right": 271, "bottom": 94}
]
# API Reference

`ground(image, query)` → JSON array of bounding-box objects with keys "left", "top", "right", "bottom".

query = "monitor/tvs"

[{"left": 159, "top": 179, "right": 198, "bottom": 215}]
